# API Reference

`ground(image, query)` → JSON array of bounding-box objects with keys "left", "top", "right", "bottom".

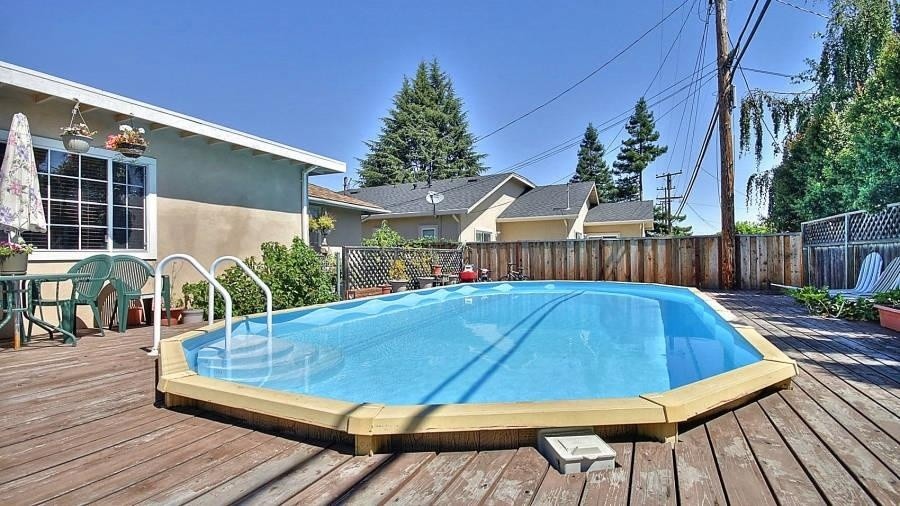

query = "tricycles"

[{"left": 475, "top": 266, "right": 493, "bottom": 282}]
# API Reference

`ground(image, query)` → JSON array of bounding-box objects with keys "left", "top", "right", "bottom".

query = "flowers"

[
  {"left": 104, "top": 123, "right": 149, "bottom": 149},
  {"left": 1, "top": 240, "right": 36, "bottom": 255},
  {"left": 60, "top": 123, "right": 94, "bottom": 136}
]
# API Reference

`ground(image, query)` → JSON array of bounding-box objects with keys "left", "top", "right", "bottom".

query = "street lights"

[{"left": 343, "top": 175, "right": 348, "bottom": 195}]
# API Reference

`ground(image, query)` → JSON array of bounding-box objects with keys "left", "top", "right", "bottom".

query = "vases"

[
  {"left": 118, "top": 140, "right": 146, "bottom": 159},
  {"left": 180, "top": 306, "right": 204, "bottom": 326},
  {"left": 0, "top": 251, "right": 28, "bottom": 275},
  {"left": 61, "top": 136, "right": 93, "bottom": 153}
]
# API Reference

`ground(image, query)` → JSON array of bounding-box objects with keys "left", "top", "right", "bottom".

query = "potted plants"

[
  {"left": 388, "top": 259, "right": 408, "bottom": 292},
  {"left": 413, "top": 249, "right": 442, "bottom": 289},
  {"left": 161, "top": 260, "right": 182, "bottom": 325},
  {"left": 27, "top": 254, "right": 171, "bottom": 347},
  {"left": 317, "top": 215, "right": 334, "bottom": 245},
  {"left": 874, "top": 289, "right": 900, "bottom": 333}
]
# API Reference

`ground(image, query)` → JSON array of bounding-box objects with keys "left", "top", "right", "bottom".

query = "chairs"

[{"left": 798, "top": 252, "right": 899, "bottom": 318}]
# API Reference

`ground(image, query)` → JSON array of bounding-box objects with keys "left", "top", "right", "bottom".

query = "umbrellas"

[{"left": 0, "top": 112, "right": 50, "bottom": 350}]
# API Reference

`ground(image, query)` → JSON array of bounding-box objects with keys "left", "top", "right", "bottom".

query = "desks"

[{"left": 0, "top": 273, "right": 91, "bottom": 347}]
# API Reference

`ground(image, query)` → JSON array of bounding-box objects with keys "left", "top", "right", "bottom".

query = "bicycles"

[{"left": 497, "top": 262, "right": 529, "bottom": 280}]
